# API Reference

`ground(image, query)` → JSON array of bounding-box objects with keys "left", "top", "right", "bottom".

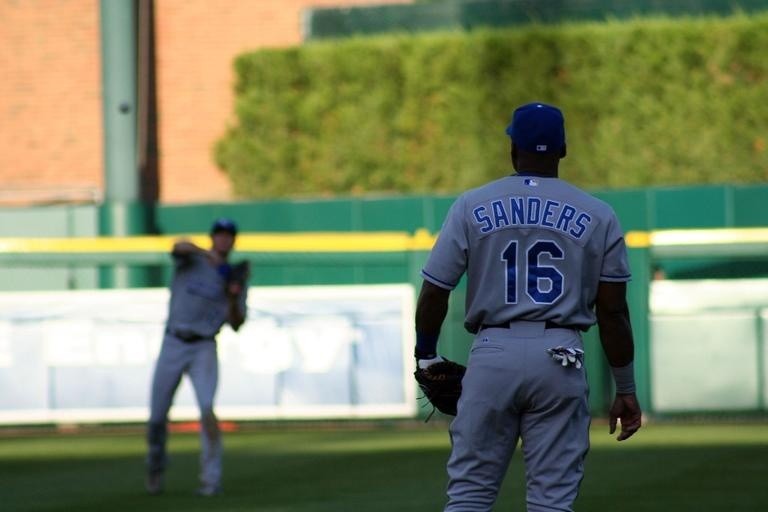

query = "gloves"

[{"left": 547, "top": 345, "right": 588, "bottom": 371}]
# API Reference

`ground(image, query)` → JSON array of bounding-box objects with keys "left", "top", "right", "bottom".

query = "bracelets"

[{"left": 609, "top": 360, "right": 636, "bottom": 394}]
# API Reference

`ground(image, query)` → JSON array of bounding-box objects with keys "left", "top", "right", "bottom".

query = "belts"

[
  {"left": 166, "top": 327, "right": 216, "bottom": 344},
  {"left": 481, "top": 322, "right": 584, "bottom": 334}
]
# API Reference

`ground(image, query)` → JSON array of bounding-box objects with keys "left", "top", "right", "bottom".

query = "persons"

[
  {"left": 145, "top": 217, "right": 249, "bottom": 496},
  {"left": 414, "top": 102, "right": 641, "bottom": 512}
]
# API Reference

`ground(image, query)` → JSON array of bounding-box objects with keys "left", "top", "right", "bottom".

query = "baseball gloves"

[
  {"left": 414, "top": 356, "right": 466, "bottom": 416},
  {"left": 224, "top": 258, "right": 249, "bottom": 298}
]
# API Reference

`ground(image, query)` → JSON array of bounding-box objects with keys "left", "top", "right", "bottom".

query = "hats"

[
  {"left": 504, "top": 102, "right": 567, "bottom": 150},
  {"left": 210, "top": 217, "right": 237, "bottom": 236}
]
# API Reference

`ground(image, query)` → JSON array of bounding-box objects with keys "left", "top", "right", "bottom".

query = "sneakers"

[
  {"left": 196, "top": 478, "right": 220, "bottom": 496},
  {"left": 147, "top": 471, "right": 164, "bottom": 495}
]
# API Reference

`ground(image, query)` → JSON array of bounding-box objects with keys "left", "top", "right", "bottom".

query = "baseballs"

[{"left": 119, "top": 103, "right": 129, "bottom": 113}]
